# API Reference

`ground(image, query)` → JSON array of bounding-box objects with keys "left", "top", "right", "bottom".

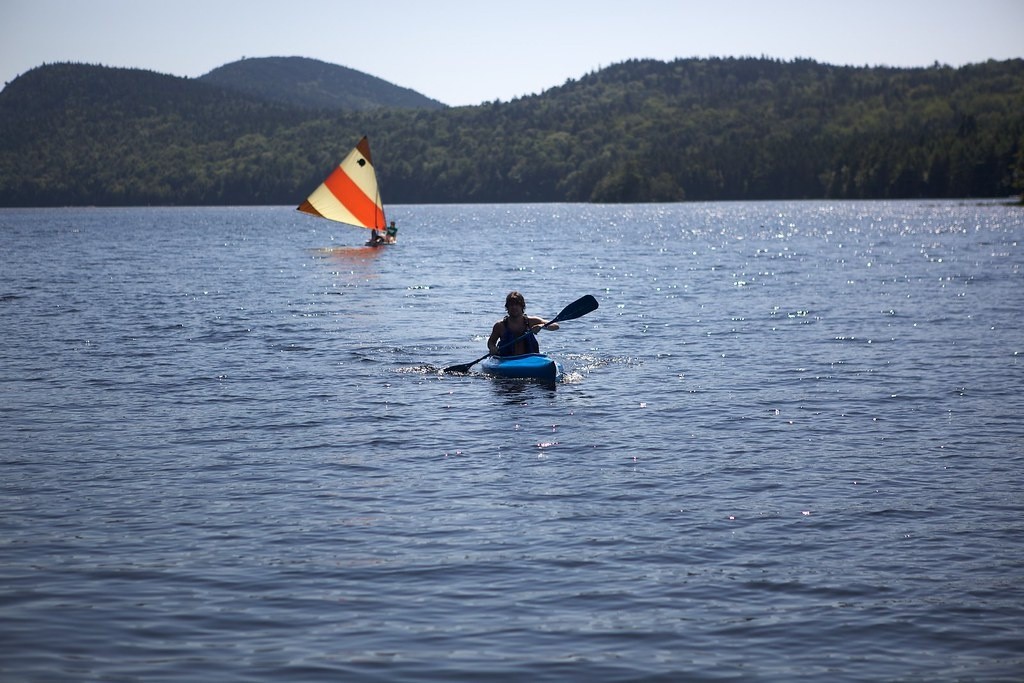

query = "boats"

[{"left": 481, "top": 353, "right": 566, "bottom": 381}]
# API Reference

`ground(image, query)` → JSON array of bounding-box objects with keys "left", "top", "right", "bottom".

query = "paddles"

[{"left": 443, "top": 293, "right": 600, "bottom": 373}]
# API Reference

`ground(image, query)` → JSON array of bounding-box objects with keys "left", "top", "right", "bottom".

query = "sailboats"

[{"left": 296, "top": 133, "right": 397, "bottom": 245}]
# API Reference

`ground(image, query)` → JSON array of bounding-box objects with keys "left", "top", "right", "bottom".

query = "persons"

[
  {"left": 487, "top": 292, "right": 560, "bottom": 356},
  {"left": 385, "top": 221, "right": 398, "bottom": 240}
]
